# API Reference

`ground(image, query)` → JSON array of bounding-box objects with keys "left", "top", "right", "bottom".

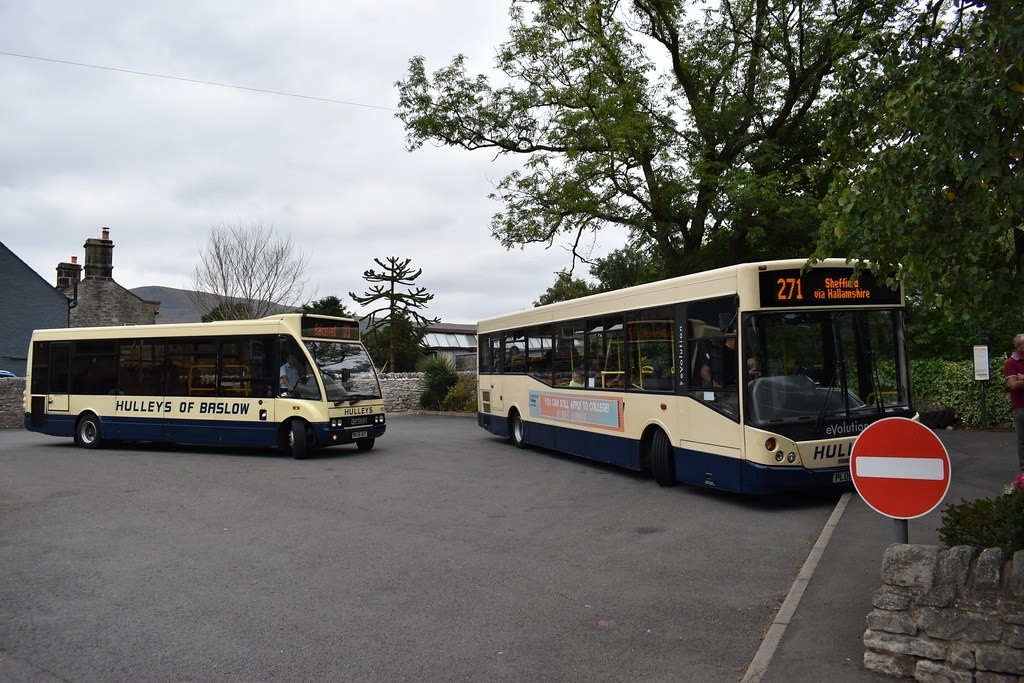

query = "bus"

[
  {"left": 22, "top": 312, "right": 387, "bottom": 460},
  {"left": 476, "top": 257, "right": 920, "bottom": 497}
]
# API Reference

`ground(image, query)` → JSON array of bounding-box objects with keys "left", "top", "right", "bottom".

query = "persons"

[
  {"left": 589, "top": 342, "right": 623, "bottom": 388},
  {"left": 504, "top": 346, "right": 519, "bottom": 371},
  {"left": 280, "top": 353, "right": 300, "bottom": 394},
  {"left": 569, "top": 367, "right": 584, "bottom": 387},
  {"left": 701, "top": 337, "right": 761, "bottom": 401},
  {"left": 1004, "top": 334, "right": 1024, "bottom": 472}
]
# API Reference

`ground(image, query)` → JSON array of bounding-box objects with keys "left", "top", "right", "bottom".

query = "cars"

[{"left": 814, "top": 387, "right": 866, "bottom": 417}]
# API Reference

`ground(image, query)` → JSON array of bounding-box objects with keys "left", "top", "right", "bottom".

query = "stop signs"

[{"left": 849, "top": 417, "right": 952, "bottom": 520}]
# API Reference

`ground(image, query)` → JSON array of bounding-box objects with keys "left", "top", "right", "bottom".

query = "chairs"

[{"left": 510, "top": 354, "right": 547, "bottom": 372}]
